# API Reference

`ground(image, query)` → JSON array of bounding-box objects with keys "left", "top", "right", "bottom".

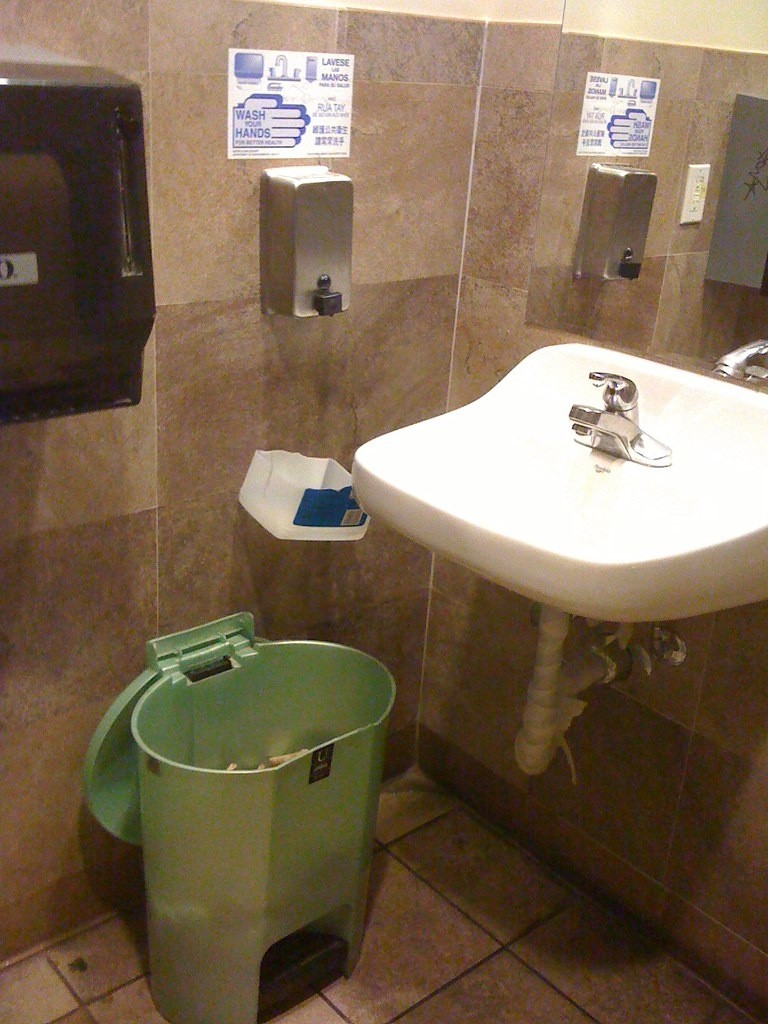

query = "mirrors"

[{"left": 523, "top": 0, "right": 768, "bottom": 394}]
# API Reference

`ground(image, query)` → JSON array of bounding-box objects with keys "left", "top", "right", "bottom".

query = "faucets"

[
  {"left": 568, "top": 369, "right": 675, "bottom": 468},
  {"left": 710, "top": 337, "right": 768, "bottom": 383}
]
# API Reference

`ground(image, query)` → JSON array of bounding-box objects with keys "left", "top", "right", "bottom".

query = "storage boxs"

[{"left": 238, "top": 451, "right": 370, "bottom": 541}]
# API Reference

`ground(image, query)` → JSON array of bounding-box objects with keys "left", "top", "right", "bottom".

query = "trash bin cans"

[{"left": 82, "top": 612, "right": 395, "bottom": 1024}]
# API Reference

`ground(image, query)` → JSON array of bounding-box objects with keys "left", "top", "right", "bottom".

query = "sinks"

[{"left": 350, "top": 344, "right": 768, "bottom": 625}]
